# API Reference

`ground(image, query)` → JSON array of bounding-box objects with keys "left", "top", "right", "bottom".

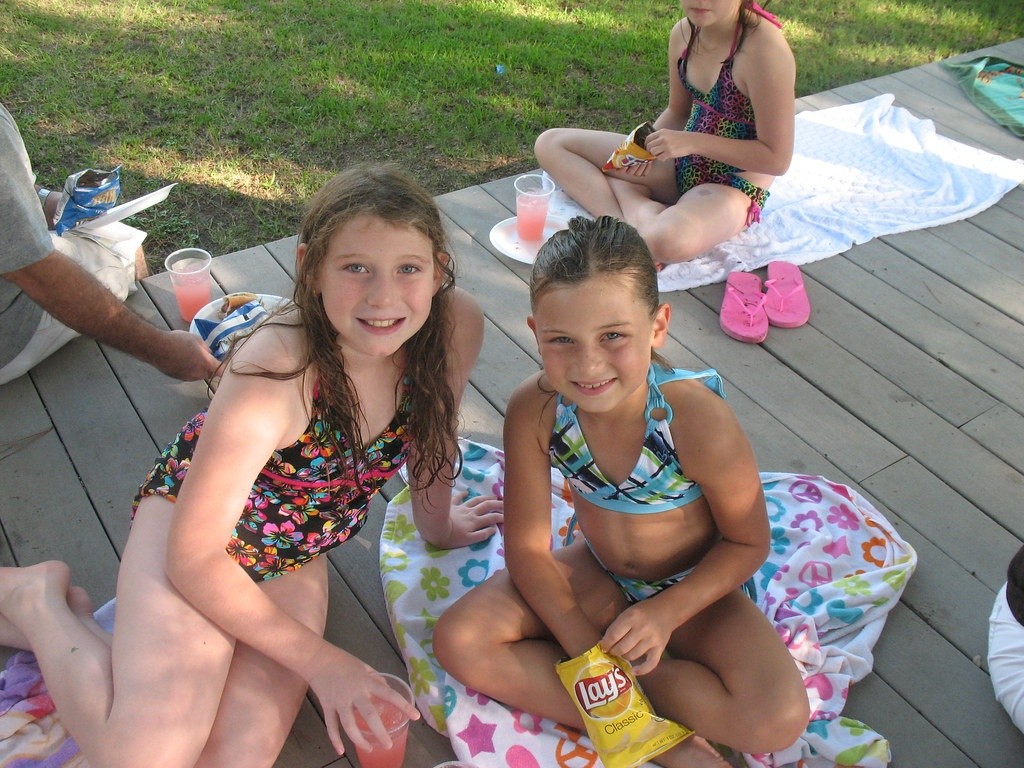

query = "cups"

[
  {"left": 165, "top": 248, "right": 211, "bottom": 324},
  {"left": 431, "top": 761, "right": 479, "bottom": 768},
  {"left": 349, "top": 672, "right": 415, "bottom": 768},
  {"left": 514, "top": 175, "right": 555, "bottom": 241}
]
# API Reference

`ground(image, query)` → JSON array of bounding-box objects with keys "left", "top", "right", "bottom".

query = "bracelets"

[{"left": 37, "top": 188, "right": 51, "bottom": 209}]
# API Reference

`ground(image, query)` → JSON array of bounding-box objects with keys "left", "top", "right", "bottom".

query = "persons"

[
  {"left": 0, "top": 101, "right": 226, "bottom": 387},
  {"left": 987, "top": 546, "right": 1024, "bottom": 735},
  {"left": 534, "top": 0, "right": 796, "bottom": 273},
  {"left": 0, "top": 162, "right": 502, "bottom": 768},
  {"left": 432, "top": 216, "right": 810, "bottom": 768}
]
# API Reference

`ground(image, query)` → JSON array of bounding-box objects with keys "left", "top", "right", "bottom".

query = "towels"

[
  {"left": 540, "top": 92, "right": 1024, "bottom": 295},
  {"left": 380, "top": 441, "right": 917, "bottom": 767},
  {"left": 0, "top": 600, "right": 120, "bottom": 768}
]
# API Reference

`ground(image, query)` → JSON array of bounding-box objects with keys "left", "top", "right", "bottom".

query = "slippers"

[
  {"left": 719, "top": 271, "right": 769, "bottom": 343},
  {"left": 764, "top": 261, "right": 811, "bottom": 329}
]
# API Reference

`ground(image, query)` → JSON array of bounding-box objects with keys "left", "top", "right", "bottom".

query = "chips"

[{"left": 602, "top": 719, "right": 668, "bottom": 753}]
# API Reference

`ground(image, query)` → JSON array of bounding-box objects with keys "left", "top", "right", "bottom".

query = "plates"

[{"left": 489, "top": 216, "right": 568, "bottom": 264}]
